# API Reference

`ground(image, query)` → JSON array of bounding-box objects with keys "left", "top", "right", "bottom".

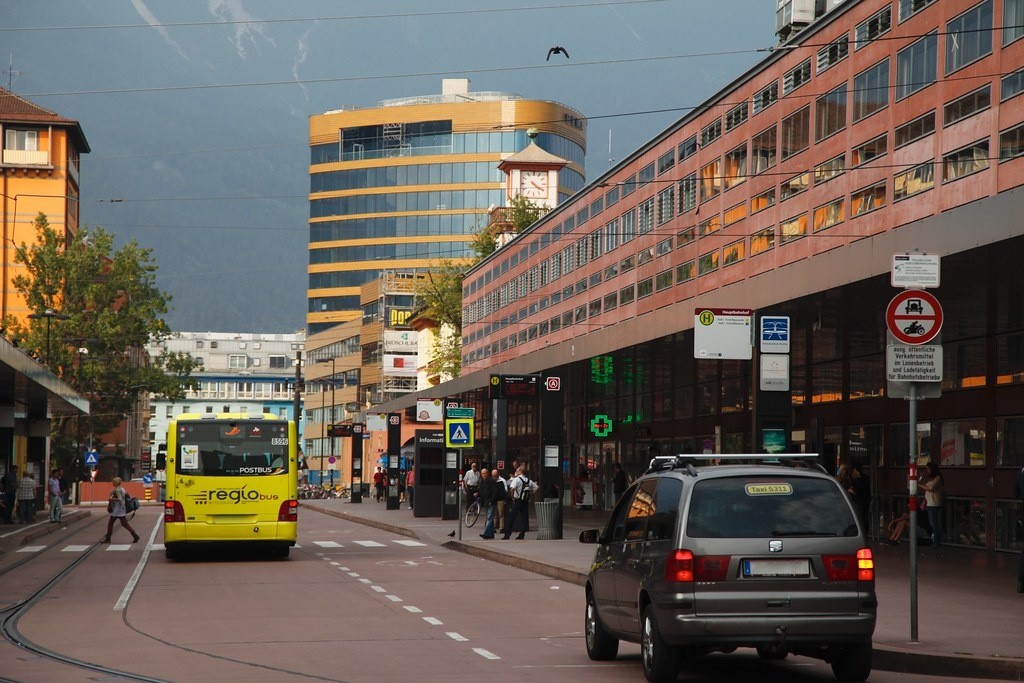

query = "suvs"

[{"left": 578, "top": 453, "right": 877, "bottom": 683}]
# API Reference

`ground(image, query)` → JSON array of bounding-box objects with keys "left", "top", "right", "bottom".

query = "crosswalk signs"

[
  {"left": 449, "top": 423, "right": 470, "bottom": 445},
  {"left": 85, "top": 452, "right": 98, "bottom": 465}
]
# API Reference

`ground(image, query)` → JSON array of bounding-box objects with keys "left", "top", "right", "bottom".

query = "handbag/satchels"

[
  {"left": 920, "top": 498, "right": 927, "bottom": 510},
  {"left": 374, "top": 483, "right": 377, "bottom": 487},
  {"left": 107, "top": 503, "right": 113, "bottom": 513}
]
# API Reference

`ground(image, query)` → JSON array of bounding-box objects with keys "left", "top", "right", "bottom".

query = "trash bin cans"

[{"left": 534, "top": 502, "right": 560, "bottom": 540}]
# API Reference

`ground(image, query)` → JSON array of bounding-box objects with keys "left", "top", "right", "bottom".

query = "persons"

[
  {"left": 400, "top": 466, "right": 414, "bottom": 509},
  {"left": 887, "top": 504, "right": 933, "bottom": 545},
  {"left": 479, "top": 461, "right": 539, "bottom": 540},
  {"left": 917, "top": 462, "right": 944, "bottom": 547},
  {"left": 579, "top": 464, "right": 588, "bottom": 478},
  {"left": 49, "top": 470, "right": 68, "bottom": 522},
  {"left": 374, "top": 467, "right": 387, "bottom": 502},
  {"left": 4, "top": 465, "right": 37, "bottom": 523},
  {"left": 463, "top": 463, "right": 482, "bottom": 515},
  {"left": 101, "top": 477, "right": 140, "bottom": 543},
  {"left": 1015, "top": 466, "right": 1024, "bottom": 592},
  {"left": 835, "top": 464, "right": 871, "bottom": 535},
  {"left": 610, "top": 463, "right": 627, "bottom": 502}
]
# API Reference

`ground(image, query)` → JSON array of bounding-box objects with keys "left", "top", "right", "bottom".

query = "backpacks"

[
  {"left": 516, "top": 477, "right": 531, "bottom": 502},
  {"left": 124, "top": 492, "right": 139, "bottom": 513}
]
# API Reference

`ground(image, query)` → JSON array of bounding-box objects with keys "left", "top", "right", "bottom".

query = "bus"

[{"left": 156, "top": 413, "right": 298, "bottom": 559}]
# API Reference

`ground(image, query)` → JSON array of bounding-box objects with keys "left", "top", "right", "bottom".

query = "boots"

[
  {"left": 101, "top": 535, "right": 111, "bottom": 542},
  {"left": 132, "top": 532, "right": 140, "bottom": 543}
]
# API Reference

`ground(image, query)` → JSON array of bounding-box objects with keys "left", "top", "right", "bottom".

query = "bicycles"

[{"left": 465, "top": 493, "right": 480, "bottom": 528}]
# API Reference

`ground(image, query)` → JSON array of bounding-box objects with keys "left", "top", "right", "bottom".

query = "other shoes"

[
  {"left": 1017, "top": 581, "right": 1024, "bottom": 592},
  {"left": 399, "top": 500, "right": 402, "bottom": 503},
  {"left": 56, "top": 520, "right": 61, "bottom": 522},
  {"left": 378, "top": 500, "right": 379, "bottom": 502},
  {"left": 501, "top": 536, "right": 510, "bottom": 540},
  {"left": 7, "top": 521, "right": 15, "bottom": 524},
  {"left": 479, "top": 534, "right": 485, "bottom": 538},
  {"left": 516, "top": 534, "right": 524, "bottom": 540},
  {"left": 51, "top": 521, "right": 55, "bottom": 523},
  {"left": 408, "top": 506, "right": 412, "bottom": 510},
  {"left": 483, "top": 536, "right": 493, "bottom": 539},
  {"left": 880, "top": 541, "right": 887, "bottom": 546},
  {"left": 402, "top": 499, "right": 406, "bottom": 502},
  {"left": 886, "top": 541, "right": 900, "bottom": 546}
]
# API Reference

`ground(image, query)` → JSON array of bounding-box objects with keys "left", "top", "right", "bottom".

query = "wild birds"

[
  {"left": 448, "top": 530, "right": 456, "bottom": 537},
  {"left": 547, "top": 47, "right": 570, "bottom": 61}
]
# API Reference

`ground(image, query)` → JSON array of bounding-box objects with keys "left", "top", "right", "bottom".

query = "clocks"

[{"left": 519, "top": 168, "right": 549, "bottom": 201}]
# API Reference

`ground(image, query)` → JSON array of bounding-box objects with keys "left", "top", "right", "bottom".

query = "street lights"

[
  {"left": 61, "top": 338, "right": 99, "bottom": 505},
  {"left": 316, "top": 358, "right": 335, "bottom": 487},
  {"left": 26, "top": 308, "right": 71, "bottom": 509}
]
